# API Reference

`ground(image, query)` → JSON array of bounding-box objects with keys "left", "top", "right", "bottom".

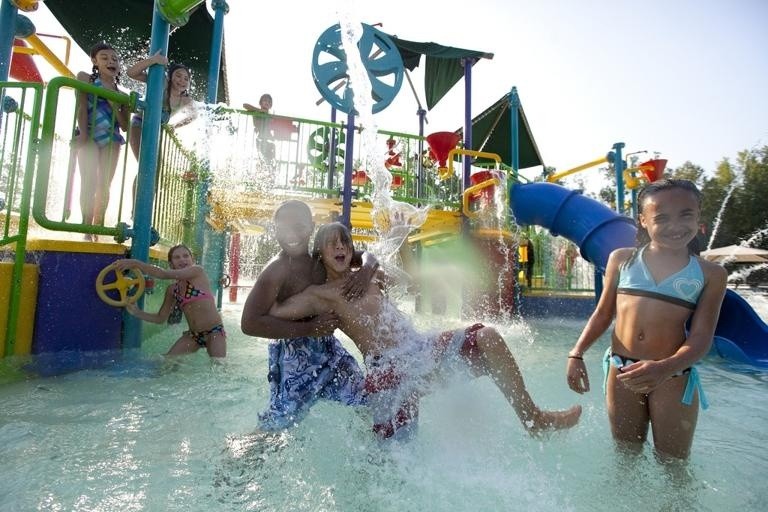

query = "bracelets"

[{"left": 568, "top": 356, "right": 583, "bottom": 360}]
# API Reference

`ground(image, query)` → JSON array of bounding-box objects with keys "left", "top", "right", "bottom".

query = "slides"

[{"left": 511, "top": 183, "right": 764, "bottom": 368}]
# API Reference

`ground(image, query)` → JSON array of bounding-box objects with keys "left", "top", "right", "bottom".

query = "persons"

[
  {"left": 126, "top": 49, "right": 199, "bottom": 220},
  {"left": 243, "top": 93, "right": 272, "bottom": 139},
  {"left": 566, "top": 178, "right": 728, "bottom": 460},
  {"left": 268, "top": 222, "right": 582, "bottom": 450},
  {"left": 241, "top": 199, "right": 379, "bottom": 430},
  {"left": 113, "top": 244, "right": 227, "bottom": 358},
  {"left": 72, "top": 44, "right": 131, "bottom": 242},
  {"left": 514, "top": 235, "right": 535, "bottom": 287}
]
